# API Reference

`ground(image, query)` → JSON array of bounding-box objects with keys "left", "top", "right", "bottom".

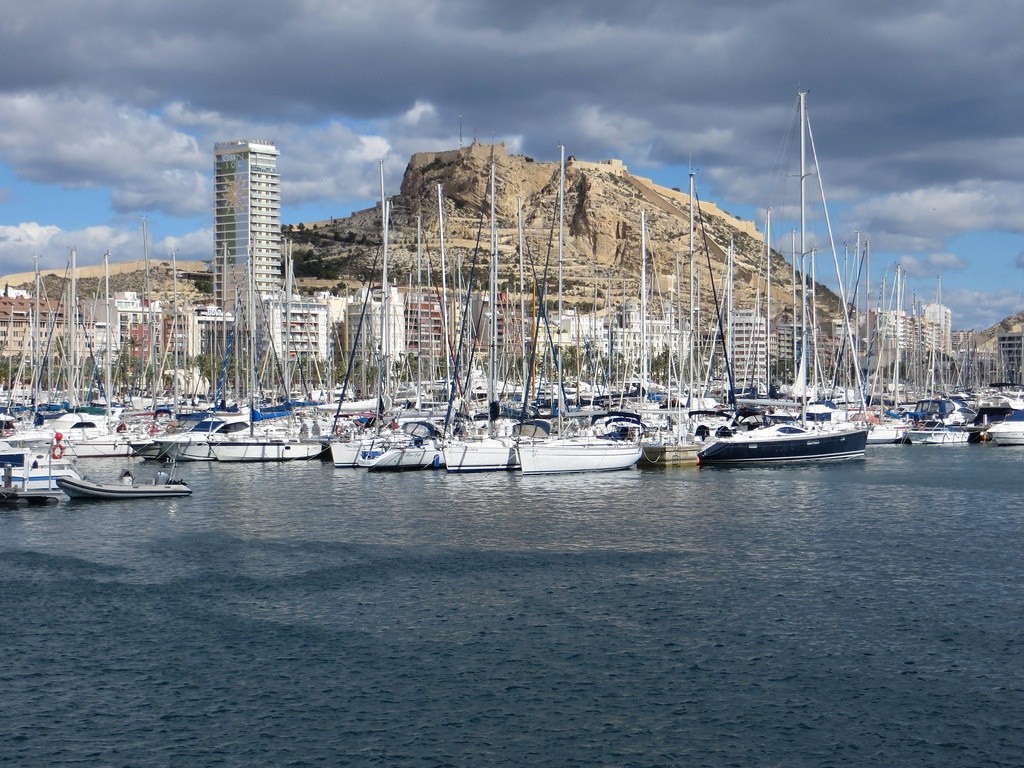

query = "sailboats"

[{"left": 1, "top": 88, "right": 1024, "bottom": 503}]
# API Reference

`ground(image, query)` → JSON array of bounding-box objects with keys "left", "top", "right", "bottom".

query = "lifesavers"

[
  {"left": 165, "top": 423, "right": 176, "bottom": 435},
  {"left": 907, "top": 420, "right": 914, "bottom": 425},
  {"left": 917, "top": 423, "right": 925, "bottom": 431},
  {"left": 51, "top": 443, "right": 64, "bottom": 460}
]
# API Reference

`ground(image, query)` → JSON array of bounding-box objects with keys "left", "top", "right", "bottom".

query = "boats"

[{"left": 55, "top": 462, "right": 194, "bottom": 498}]
